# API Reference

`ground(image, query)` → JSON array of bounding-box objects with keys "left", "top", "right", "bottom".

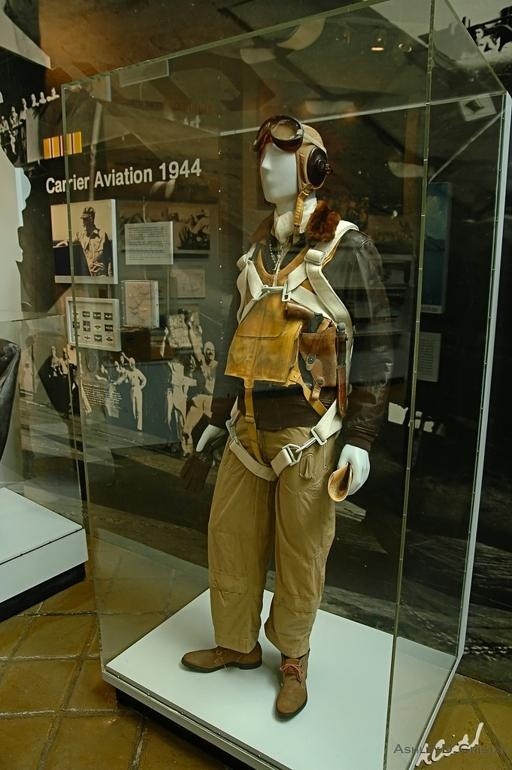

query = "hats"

[{"left": 79, "top": 206, "right": 95, "bottom": 220}]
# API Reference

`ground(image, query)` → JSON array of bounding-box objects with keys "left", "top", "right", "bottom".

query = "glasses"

[{"left": 251, "top": 114, "right": 304, "bottom": 155}]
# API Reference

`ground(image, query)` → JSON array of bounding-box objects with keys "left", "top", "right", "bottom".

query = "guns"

[{"left": 286, "top": 302, "right": 324, "bottom": 333}]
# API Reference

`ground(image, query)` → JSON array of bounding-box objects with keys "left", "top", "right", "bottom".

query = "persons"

[
  {"left": 179, "top": 313, "right": 221, "bottom": 456},
  {"left": 46, "top": 342, "right": 148, "bottom": 435},
  {"left": 180, "top": 114, "right": 397, "bottom": 719},
  {"left": 57, "top": 204, "right": 109, "bottom": 277},
  {"left": 158, "top": 325, "right": 189, "bottom": 454}
]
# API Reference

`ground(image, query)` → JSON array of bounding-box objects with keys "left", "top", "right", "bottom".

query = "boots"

[
  {"left": 182, "top": 642, "right": 263, "bottom": 672},
  {"left": 275, "top": 649, "right": 309, "bottom": 719}
]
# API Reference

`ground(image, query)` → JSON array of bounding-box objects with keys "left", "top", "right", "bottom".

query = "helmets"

[{"left": 250, "top": 121, "right": 326, "bottom": 193}]
